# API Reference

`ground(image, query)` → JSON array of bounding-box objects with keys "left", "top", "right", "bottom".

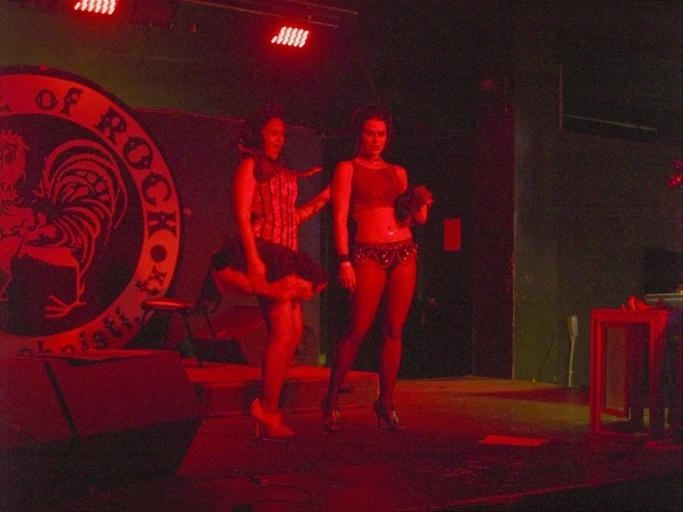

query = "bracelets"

[{"left": 339, "top": 254, "right": 351, "bottom": 265}]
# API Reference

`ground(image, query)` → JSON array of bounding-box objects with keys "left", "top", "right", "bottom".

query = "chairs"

[{"left": 137, "top": 250, "right": 224, "bottom": 369}]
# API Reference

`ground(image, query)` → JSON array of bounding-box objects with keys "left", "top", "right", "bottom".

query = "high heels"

[
  {"left": 374, "top": 399, "right": 406, "bottom": 431},
  {"left": 251, "top": 398, "right": 296, "bottom": 438},
  {"left": 320, "top": 397, "right": 338, "bottom": 430}
]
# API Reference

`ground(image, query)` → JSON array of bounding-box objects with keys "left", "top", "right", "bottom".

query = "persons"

[
  {"left": 208, "top": 110, "right": 331, "bottom": 438},
  {"left": 321, "top": 95, "right": 434, "bottom": 436}
]
